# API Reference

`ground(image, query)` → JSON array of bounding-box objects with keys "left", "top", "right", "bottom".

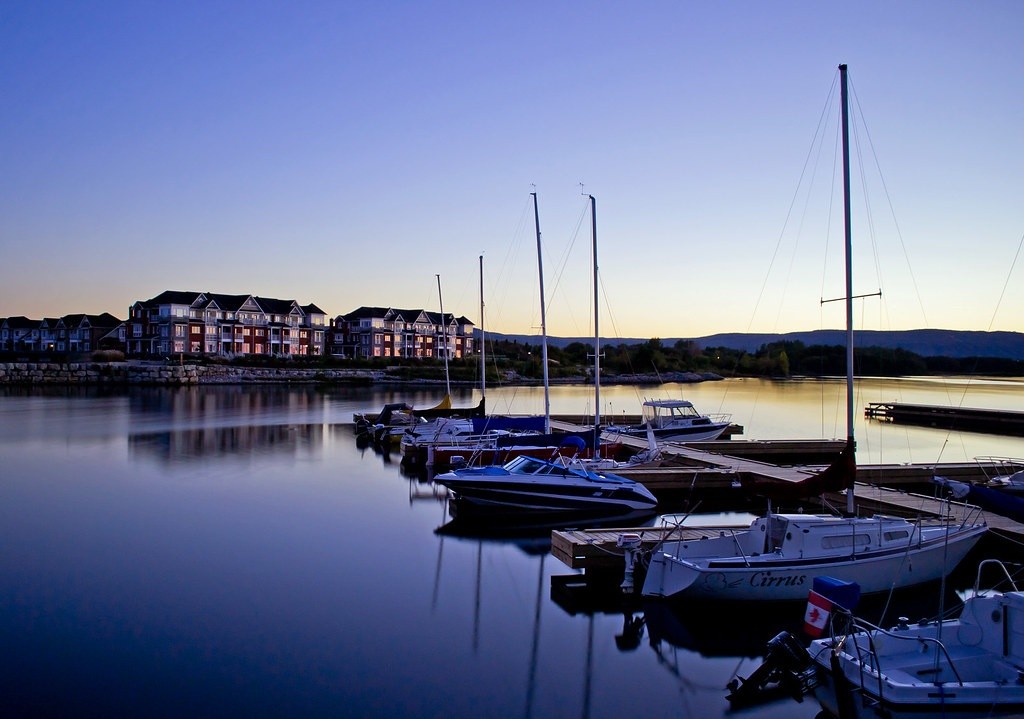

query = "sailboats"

[
  {"left": 613, "top": 60, "right": 991, "bottom": 605},
  {"left": 724, "top": 235, "right": 1023, "bottom": 719},
  {"left": 399, "top": 254, "right": 513, "bottom": 463},
  {"left": 352, "top": 271, "right": 452, "bottom": 449},
  {"left": 476, "top": 184, "right": 558, "bottom": 435},
  {"left": 547, "top": 181, "right": 667, "bottom": 468}
]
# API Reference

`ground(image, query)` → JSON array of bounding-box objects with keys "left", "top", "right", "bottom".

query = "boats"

[
  {"left": 604, "top": 397, "right": 733, "bottom": 444},
  {"left": 432, "top": 435, "right": 659, "bottom": 513}
]
